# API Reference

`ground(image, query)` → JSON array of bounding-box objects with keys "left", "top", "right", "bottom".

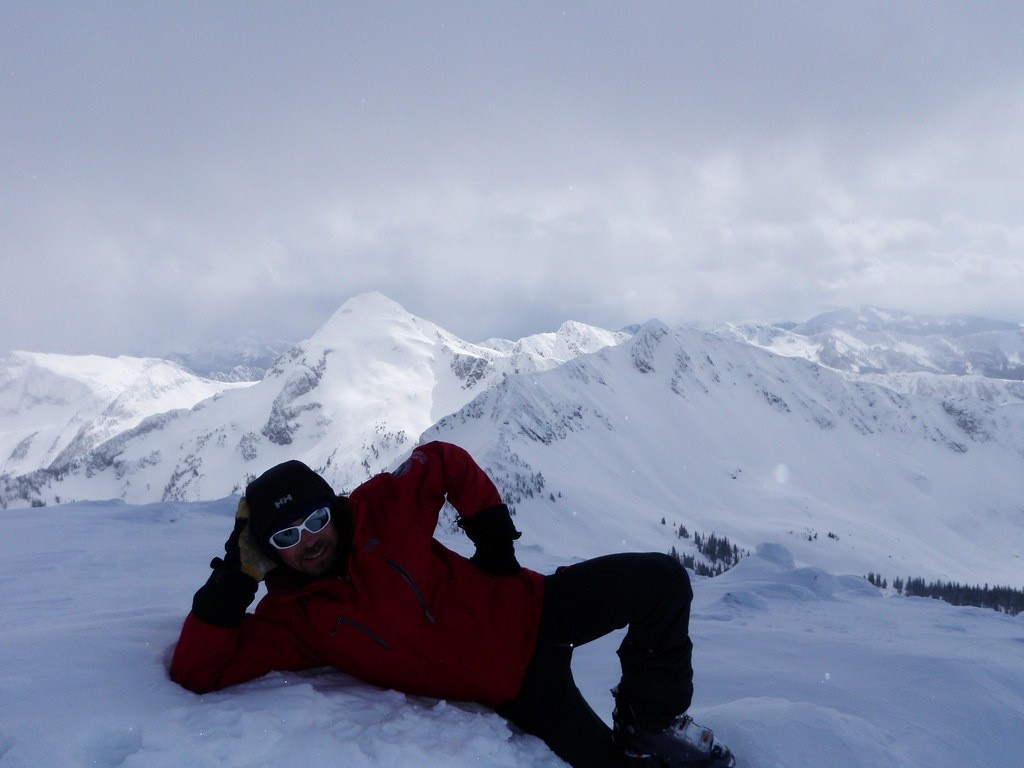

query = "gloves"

[
  {"left": 457, "top": 503, "right": 524, "bottom": 577},
  {"left": 211, "top": 495, "right": 288, "bottom": 581}
]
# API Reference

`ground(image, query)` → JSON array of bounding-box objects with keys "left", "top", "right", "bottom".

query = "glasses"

[{"left": 269, "top": 506, "right": 331, "bottom": 550}]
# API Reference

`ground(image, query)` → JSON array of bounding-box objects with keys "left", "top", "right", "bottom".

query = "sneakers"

[{"left": 612, "top": 684, "right": 736, "bottom": 768}]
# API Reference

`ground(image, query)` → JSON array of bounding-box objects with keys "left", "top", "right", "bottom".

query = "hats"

[{"left": 246, "top": 459, "right": 335, "bottom": 547}]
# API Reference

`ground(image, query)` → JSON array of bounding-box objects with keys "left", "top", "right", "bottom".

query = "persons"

[{"left": 168, "top": 440, "right": 737, "bottom": 768}]
